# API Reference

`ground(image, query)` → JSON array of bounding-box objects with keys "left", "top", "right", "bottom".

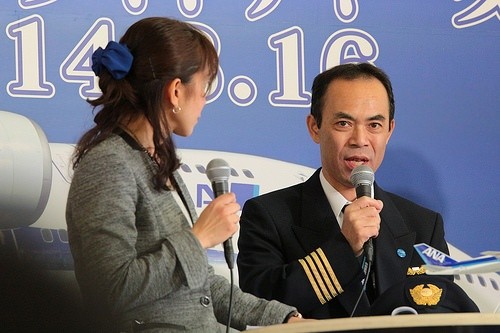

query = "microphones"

[
  {"left": 206, "top": 158, "right": 235, "bottom": 269},
  {"left": 350, "top": 165, "right": 375, "bottom": 262}
]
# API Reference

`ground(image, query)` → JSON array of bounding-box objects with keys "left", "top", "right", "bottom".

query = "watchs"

[{"left": 285, "top": 309, "right": 302, "bottom": 321}]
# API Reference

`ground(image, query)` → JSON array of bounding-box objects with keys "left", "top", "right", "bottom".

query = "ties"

[{"left": 341, "top": 204, "right": 378, "bottom": 307}]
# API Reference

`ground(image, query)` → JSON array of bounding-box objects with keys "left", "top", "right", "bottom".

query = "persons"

[
  {"left": 237, "top": 62, "right": 479, "bottom": 321},
  {"left": 65, "top": 17, "right": 322, "bottom": 333}
]
